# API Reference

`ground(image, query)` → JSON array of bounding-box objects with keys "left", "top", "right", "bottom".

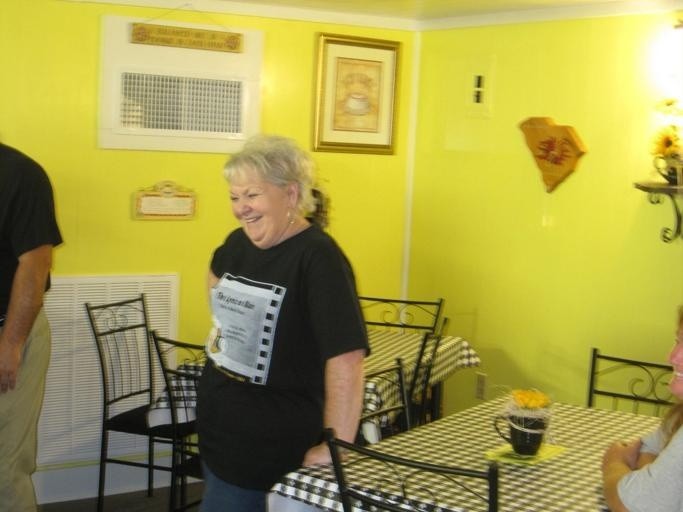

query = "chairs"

[
  {"left": 150, "top": 327, "right": 221, "bottom": 510},
  {"left": 586, "top": 344, "right": 673, "bottom": 420},
  {"left": 407, "top": 317, "right": 452, "bottom": 421},
  {"left": 351, "top": 294, "right": 446, "bottom": 335},
  {"left": 321, "top": 428, "right": 502, "bottom": 511},
  {"left": 338, "top": 357, "right": 417, "bottom": 446},
  {"left": 85, "top": 294, "right": 198, "bottom": 506}
]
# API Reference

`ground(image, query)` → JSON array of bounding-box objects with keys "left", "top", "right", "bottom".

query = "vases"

[{"left": 654, "top": 156, "right": 682, "bottom": 185}]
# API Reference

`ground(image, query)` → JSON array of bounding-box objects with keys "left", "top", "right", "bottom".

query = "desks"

[
  {"left": 148, "top": 321, "right": 483, "bottom": 433},
  {"left": 264, "top": 388, "right": 671, "bottom": 509}
]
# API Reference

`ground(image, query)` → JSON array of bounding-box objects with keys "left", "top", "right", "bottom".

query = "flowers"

[{"left": 647, "top": 121, "right": 683, "bottom": 162}]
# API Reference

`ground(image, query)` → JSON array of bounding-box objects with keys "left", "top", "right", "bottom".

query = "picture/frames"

[{"left": 311, "top": 31, "right": 402, "bottom": 157}]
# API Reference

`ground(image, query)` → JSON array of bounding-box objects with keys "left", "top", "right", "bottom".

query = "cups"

[{"left": 496, "top": 403, "right": 553, "bottom": 460}]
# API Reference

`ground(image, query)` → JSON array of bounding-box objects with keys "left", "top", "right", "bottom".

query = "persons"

[
  {"left": 601, "top": 304, "right": 683, "bottom": 512},
  {"left": 195, "top": 134, "right": 372, "bottom": 511},
  {"left": 0, "top": 138, "right": 63, "bottom": 512}
]
